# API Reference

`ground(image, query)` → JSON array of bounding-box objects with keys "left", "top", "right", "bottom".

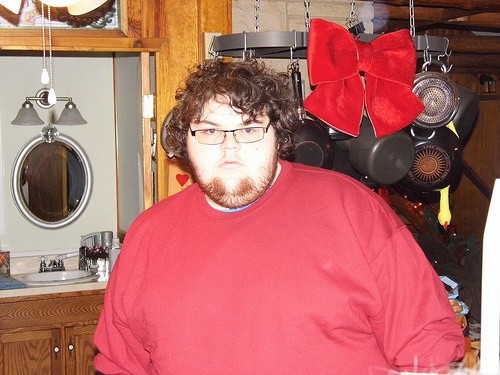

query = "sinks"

[{"left": 23, "top": 270, "right": 90, "bottom": 282}]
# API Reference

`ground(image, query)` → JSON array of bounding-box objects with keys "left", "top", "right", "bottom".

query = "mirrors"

[{"left": 10, "top": 126, "right": 93, "bottom": 229}]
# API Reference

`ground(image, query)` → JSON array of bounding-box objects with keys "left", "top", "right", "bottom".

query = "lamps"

[{"left": 11, "top": 87, "right": 87, "bottom": 126}]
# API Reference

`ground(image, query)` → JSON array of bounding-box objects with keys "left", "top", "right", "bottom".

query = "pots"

[
  {"left": 444, "top": 82, "right": 479, "bottom": 139},
  {"left": 400, "top": 125, "right": 461, "bottom": 190},
  {"left": 347, "top": 118, "right": 414, "bottom": 185},
  {"left": 291, "top": 71, "right": 334, "bottom": 169}
]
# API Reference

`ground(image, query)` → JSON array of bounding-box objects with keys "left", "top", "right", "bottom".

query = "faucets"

[{"left": 40, "top": 262, "right": 46, "bottom": 272}]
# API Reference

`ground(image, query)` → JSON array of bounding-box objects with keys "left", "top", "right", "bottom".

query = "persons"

[{"left": 92, "top": 57, "right": 466, "bottom": 375}]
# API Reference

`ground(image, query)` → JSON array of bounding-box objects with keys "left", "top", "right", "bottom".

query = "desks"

[{"left": 0, "top": 288, "right": 105, "bottom": 375}]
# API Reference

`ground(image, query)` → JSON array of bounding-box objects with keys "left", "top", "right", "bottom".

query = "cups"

[{"left": 108, "top": 249, "right": 121, "bottom": 273}]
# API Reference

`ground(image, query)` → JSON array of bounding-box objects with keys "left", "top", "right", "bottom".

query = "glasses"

[{"left": 187, "top": 120, "right": 272, "bottom": 144}]
